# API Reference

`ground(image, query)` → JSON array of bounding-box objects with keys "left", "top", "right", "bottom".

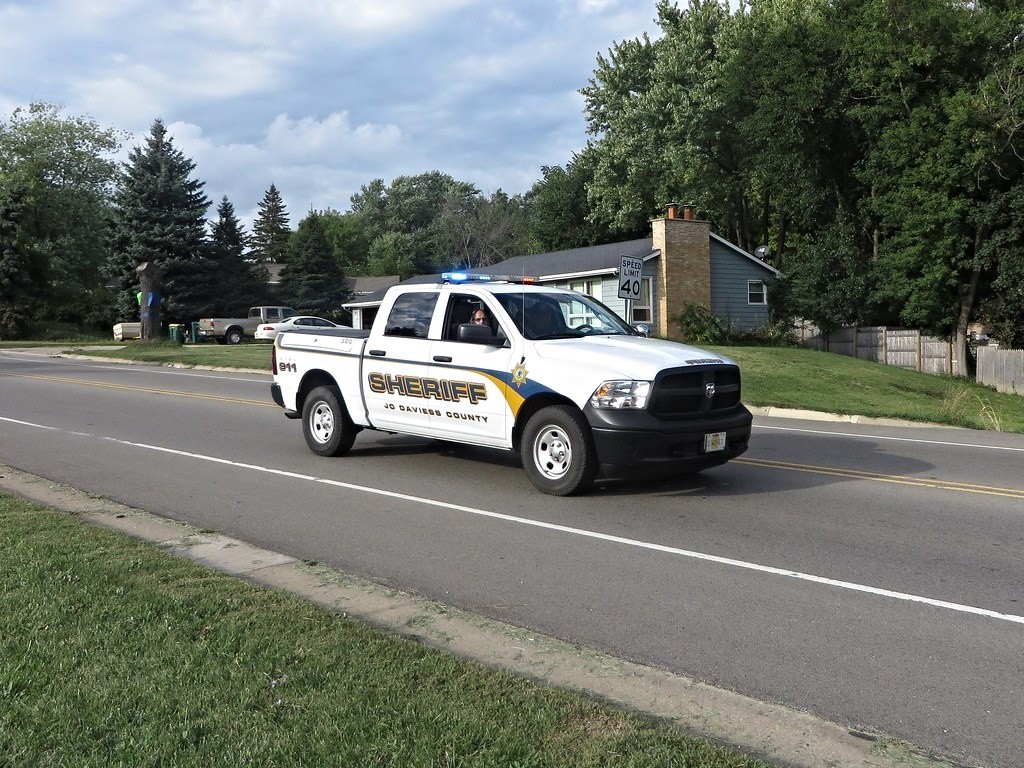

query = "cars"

[{"left": 254, "top": 316, "right": 353, "bottom": 343}]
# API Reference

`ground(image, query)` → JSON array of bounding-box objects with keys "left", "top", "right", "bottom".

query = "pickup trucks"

[
  {"left": 198, "top": 306, "right": 301, "bottom": 345},
  {"left": 271, "top": 271, "right": 753, "bottom": 497}
]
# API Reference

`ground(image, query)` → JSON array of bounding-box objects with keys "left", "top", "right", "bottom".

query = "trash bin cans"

[
  {"left": 169, "top": 323, "right": 185, "bottom": 347},
  {"left": 192, "top": 322, "right": 205, "bottom": 343}
]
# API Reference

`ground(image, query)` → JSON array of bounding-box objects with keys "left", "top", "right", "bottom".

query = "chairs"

[{"left": 529, "top": 303, "right": 561, "bottom": 337}]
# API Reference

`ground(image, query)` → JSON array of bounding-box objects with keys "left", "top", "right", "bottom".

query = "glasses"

[{"left": 473, "top": 318, "right": 487, "bottom": 322}]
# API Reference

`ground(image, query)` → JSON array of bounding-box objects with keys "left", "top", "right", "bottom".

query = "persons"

[
  {"left": 471, "top": 309, "right": 490, "bottom": 326},
  {"left": 496, "top": 297, "right": 536, "bottom": 338}
]
